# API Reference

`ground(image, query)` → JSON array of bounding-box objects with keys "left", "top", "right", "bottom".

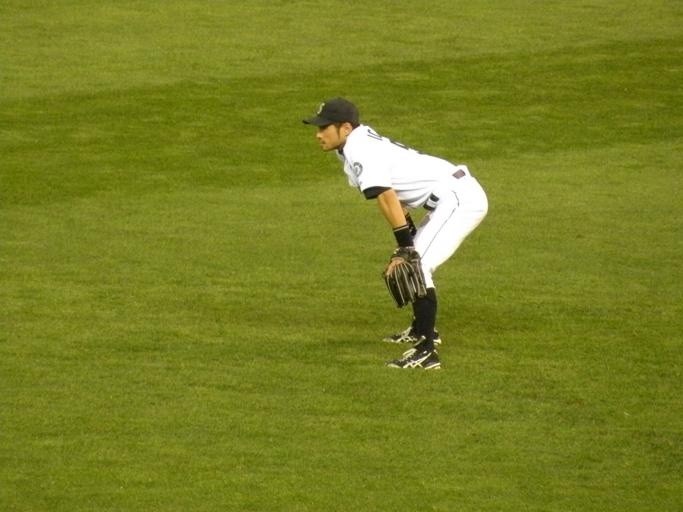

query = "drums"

[{"left": 381, "top": 245, "right": 427, "bottom": 307}]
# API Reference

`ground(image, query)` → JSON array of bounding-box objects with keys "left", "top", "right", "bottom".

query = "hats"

[{"left": 303, "top": 97, "right": 358, "bottom": 126}]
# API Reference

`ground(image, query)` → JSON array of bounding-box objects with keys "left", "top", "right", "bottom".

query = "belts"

[{"left": 452, "top": 169, "right": 466, "bottom": 178}]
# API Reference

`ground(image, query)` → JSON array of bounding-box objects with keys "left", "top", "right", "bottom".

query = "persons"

[{"left": 301, "top": 97, "right": 489, "bottom": 370}]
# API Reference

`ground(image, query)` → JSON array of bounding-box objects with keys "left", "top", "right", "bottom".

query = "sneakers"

[{"left": 382, "top": 327, "right": 441, "bottom": 369}]
